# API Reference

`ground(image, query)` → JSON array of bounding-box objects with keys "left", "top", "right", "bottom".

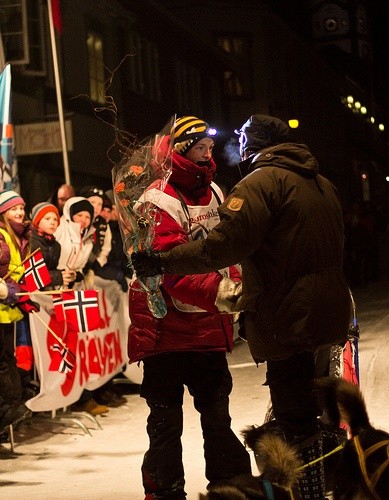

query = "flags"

[
  {"left": 0, "top": 64, "right": 21, "bottom": 200},
  {"left": 51, "top": 0, "right": 62, "bottom": 35}
]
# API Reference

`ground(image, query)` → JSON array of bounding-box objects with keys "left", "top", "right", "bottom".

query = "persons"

[
  {"left": 129, "top": 113, "right": 352, "bottom": 500},
  {"left": 52, "top": 196, "right": 110, "bottom": 415},
  {"left": 80, "top": 185, "right": 126, "bottom": 409},
  {"left": 46, "top": 184, "right": 77, "bottom": 219},
  {"left": 90, "top": 192, "right": 114, "bottom": 273},
  {"left": 0, "top": 277, "right": 31, "bottom": 428},
  {"left": 0, "top": 190, "right": 41, "bottom": 442},
  {"left": 95, "top": 189, "right": 134, "bottom": 294},
  {"left": 28, "top": 200, "right": 79, "bottom": 293},
  {"left": 126, "top": 116, "right": 254, "bottom": 499}
]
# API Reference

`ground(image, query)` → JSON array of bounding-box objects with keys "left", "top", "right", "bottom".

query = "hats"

[
  {"left": 70, "top": 200, "right": 94, "bottom": 222},
  {"left": 0, "top": 189, "right": 25, "bottom": 214},
  {"left": 170, "top": 116, "right": 215, "bottom": 154},
  {"left": 234, "top": 113, "right": 291, "bottom": 154},
  {"left": 30, "top": 202, "right": 60, "bottom": 228}
]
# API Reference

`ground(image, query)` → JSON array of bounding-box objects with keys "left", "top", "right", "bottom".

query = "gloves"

[{"left": 131, "top": 251, "right": 163, "bottom": 279}]
